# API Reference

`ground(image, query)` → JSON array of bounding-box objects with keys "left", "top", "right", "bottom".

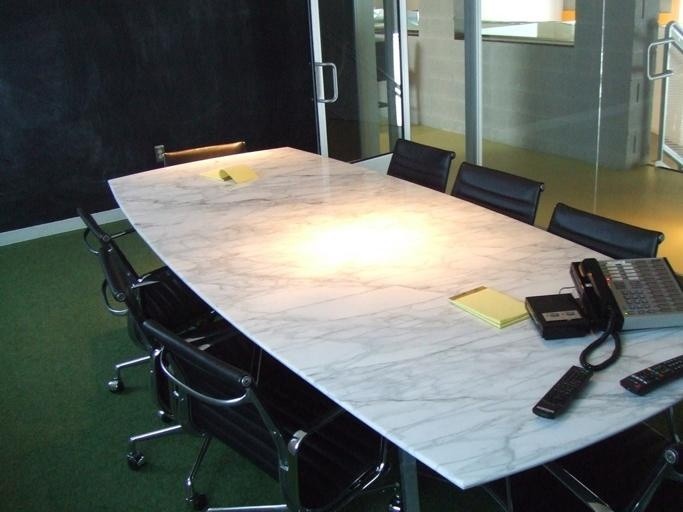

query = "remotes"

[
  {"left": 532, "top": 365, "right": 593, "bottom": 420},
  {"left": 620, "top": 354, "right": 683, "bottom": 396}
]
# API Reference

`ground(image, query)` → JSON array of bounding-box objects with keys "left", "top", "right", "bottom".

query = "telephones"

[{"left": 569, "top": 257, "right": 683, "bottom": 329}]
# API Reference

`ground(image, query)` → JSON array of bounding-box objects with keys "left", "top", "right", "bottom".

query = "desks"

[{"left": 106, "top": 145, "right": 683, "bottom": 511}]
